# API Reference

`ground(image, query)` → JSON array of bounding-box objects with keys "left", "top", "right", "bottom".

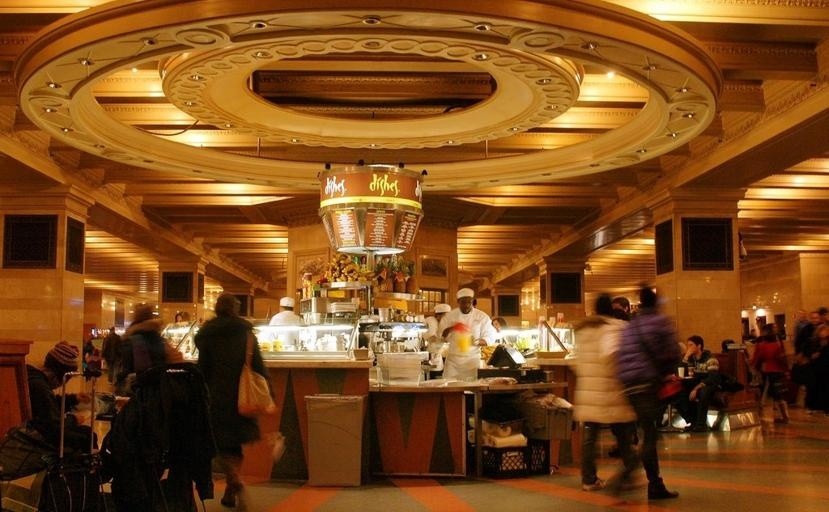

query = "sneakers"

[
  {"left": 581, "top": 477, "right": 608, "bottom": 492},
  {"left": 648, "top": 477, "right": 680, "bottom": 500}
]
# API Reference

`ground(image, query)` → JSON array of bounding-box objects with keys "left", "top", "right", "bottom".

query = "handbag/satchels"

[
  {"left": 0, "top": 426, "right": 57, "bottom": 482},
  {"left": 164, "top": 343, "right": 183, "bottom": 365},
  {"left": 658, "top": 373, "right": 683, "bottom": 399},
  {"left": 237, "top": 370, "right": 280, "bottom": 418},
  {"left": 89, "top": 390, "right": 117, "bottom": 416}
]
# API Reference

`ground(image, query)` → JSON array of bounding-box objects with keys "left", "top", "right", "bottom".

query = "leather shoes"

[{"left": 684, "top": 421, "right": 707, "bottom": 432}]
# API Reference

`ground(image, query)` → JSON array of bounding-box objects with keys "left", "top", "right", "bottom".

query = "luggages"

[{"left": 37, "top": 371, "right": 122, "bottom": 512}]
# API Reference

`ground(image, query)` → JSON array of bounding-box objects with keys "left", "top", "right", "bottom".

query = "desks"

[{"left": 369, "top": 375, "right": 569, "bottom": 479}]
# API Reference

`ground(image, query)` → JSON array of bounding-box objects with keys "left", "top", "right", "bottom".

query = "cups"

[
  {"left": 537, "top": 312, "right": 565, "bottom": 328},
  {"left": 378, "top": 308, "right": 388, "bottom": 323},
  {"left": 687, "top": 367, "right": 697, "bottom": 377},
  {"left": 678, "top": 367, "right": 684, "bottom": 378}
]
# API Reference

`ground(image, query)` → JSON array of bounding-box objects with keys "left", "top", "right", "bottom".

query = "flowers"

[{"left": 324, "top": 252, "right": 370, "bottom": 285}]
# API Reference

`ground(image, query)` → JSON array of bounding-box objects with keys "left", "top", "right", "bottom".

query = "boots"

[
  {"left": 775, "top": 404, "right": 789, "bottom": 424},
  {"left": 220, "top": 479, "right": 245, "bottom": 509}
]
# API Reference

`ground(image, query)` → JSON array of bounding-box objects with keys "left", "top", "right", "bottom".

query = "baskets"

[
  {"left": 526, "top": 438, "right": 551, "bottom": 477},
  {"left": 470, "top": 445, "right": 532, "bottom": 479}
]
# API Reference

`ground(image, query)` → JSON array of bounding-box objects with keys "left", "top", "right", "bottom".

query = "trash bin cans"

[{"left": 304, "top": 393, "right": 363, "bottom": 487}]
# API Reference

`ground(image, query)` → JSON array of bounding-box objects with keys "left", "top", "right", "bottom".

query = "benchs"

[{"left": 682, "top": 349, "right": 759, "bottom": 428}]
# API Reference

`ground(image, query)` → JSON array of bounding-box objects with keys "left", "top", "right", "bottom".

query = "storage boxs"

[{"left": 520, "top": 397, "right": 574, "bottom": 442}]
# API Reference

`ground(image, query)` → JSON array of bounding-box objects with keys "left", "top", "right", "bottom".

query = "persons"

[
  {"left": 608, "top": 296, "right": 828, "bottom": 459},
  {"left": 616, "top": 286, "right": 681, "bottom": 501},
  {"left": 195, "top": 293, "right": 276, "bottom": 511},
  {"left": 81, "top": 301, "right": 195, "bottom": 423},
  {"left": 421, "top": 287, "right": 508, "bottom": 383},
  {"left": 25, "top": 338, "right": 96, "bottom": 453},
  {"left": 570, "top": 293, "right": 644, "bottom": 490},
  {"left": 268, "top": 296, "right": 302, "bottom": 323}
]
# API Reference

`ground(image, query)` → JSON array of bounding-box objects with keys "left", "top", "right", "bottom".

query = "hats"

[
  {"left": 434, "top": 304, "right": 452, "bottom": 313},
  {"left": 280, "top": 297, "right": 296, "bottom": 308},
  {"left": 135, "top": 302, "right": 152, "bottom": 320},
  {"left": 456, "top": 288, "right": 475, "bottom": 299},
  {"left": 43, "top": 340, "right": 80, "bottom": 372}
]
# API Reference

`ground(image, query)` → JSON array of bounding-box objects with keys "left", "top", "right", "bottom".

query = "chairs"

[{"left": 660, "top": 375, "right": 686, "bottom": 432}]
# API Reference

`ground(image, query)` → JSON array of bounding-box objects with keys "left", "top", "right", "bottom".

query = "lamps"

[{"left": 738, "top": 234, "right": 748, "bottom": 261}]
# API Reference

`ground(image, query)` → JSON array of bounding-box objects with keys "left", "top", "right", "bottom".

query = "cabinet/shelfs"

[
  {"left": 494, "top": 321, "right": 577, "bottom": 359},
  {"left": 161, "top": 317, "right": 363, "bottom": 359},
  {"left": 373, "top": 293, "right": 427, "bottom": 329},
  {"left": 299, "top": 296, "right": 328, "bottom": 315}
]
